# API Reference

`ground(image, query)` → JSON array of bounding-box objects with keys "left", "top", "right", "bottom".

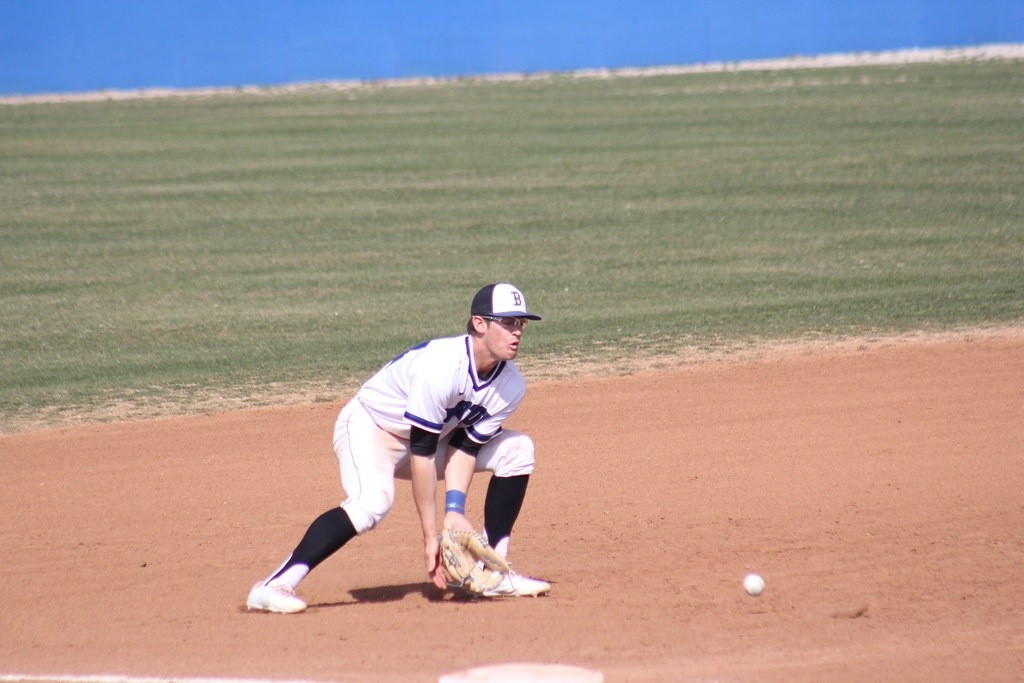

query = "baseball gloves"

[{"left": 439, "top": 528, "right": 510, "bottom": 595}]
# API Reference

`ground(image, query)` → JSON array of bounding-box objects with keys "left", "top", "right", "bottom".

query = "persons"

[{"left": 246, "top": 283, "right": 552, "bottom": 614}]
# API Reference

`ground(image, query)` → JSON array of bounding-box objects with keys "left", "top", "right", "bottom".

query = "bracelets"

[{"left": 445, "top": 490, "right": 467, "bottom": 517}]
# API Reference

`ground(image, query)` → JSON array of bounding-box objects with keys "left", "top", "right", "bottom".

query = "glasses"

[{"left": 479, "top": 316, "right": 528, "bottom": 326}]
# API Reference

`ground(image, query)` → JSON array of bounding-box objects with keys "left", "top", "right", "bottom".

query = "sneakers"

[
  {"left": 246, "top": 581, "right": 306, "bottom": 614},
  {"left": 467, "top": 565, "right": 551, "bottom": 597}
]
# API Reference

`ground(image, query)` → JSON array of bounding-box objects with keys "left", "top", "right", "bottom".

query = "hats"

[{"left": 470, "top": 283, "right": 544, "bottom": 321}]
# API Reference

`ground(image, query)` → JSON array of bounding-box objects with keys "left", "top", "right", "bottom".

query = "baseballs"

[{"left": 743, "top": 573, "right": 765, "bottom": 594}]
